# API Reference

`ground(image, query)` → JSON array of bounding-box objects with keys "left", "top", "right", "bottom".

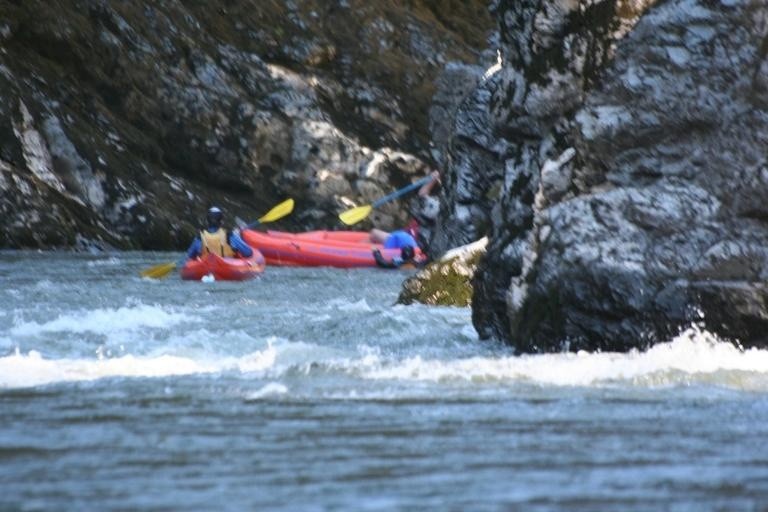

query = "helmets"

[
  {"left": 207, "top": 207, "right": 222, "bottom": 224},
  {"left": 402, "top": 245, "right": 413, "bottom": 259}
]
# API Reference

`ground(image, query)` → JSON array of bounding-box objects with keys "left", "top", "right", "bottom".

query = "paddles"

[
  {"left": 141, "top": 199, "right": 294, "bottom": 280},
  {"left": 339, "top": 174, "right": 435, "bottom": 226}
]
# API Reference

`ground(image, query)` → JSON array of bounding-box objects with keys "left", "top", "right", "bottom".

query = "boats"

[{"left": 179, "top": 226, "right": 416, "bottom": 281}]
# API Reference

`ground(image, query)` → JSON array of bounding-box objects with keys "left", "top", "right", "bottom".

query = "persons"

[
  {"left": 185, "top": 205, "right": 252, "bottom": 262},
  {"left": 369, "top": 168, "right": 441, "bottom": 248}
]
